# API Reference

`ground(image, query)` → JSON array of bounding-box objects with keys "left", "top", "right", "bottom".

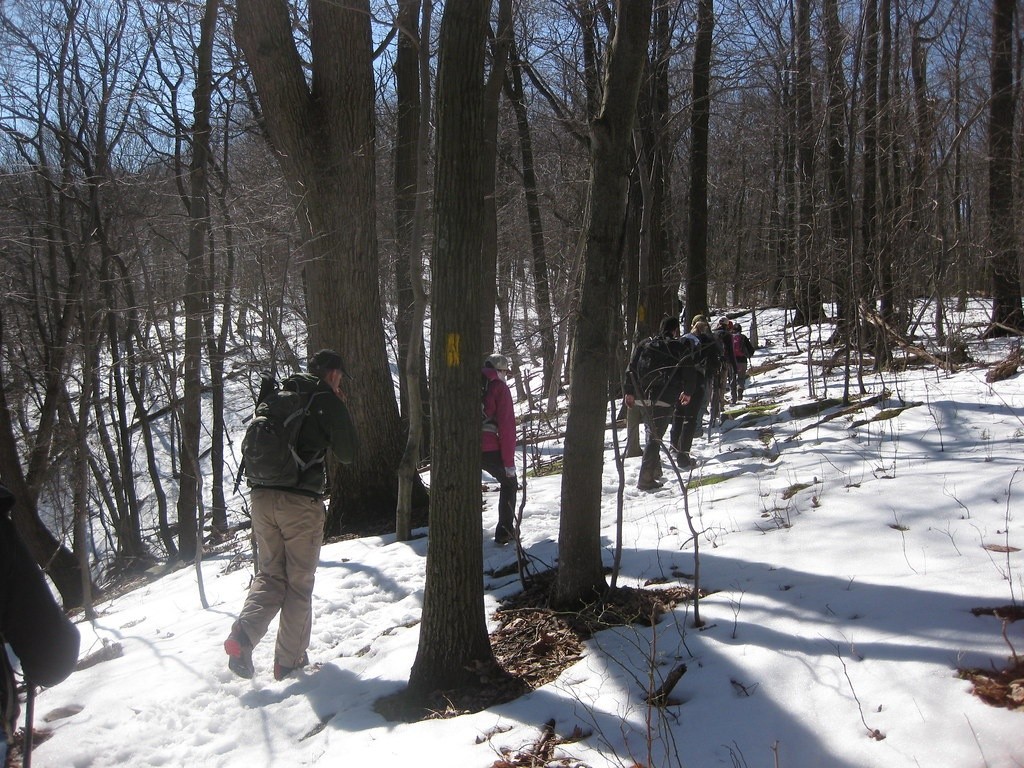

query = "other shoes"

[
  {"left": 711, "top": 418, "right": 716, "bottom": 428},
  {"left": 223, "top": 623, "right": 255, "bottom": 680},
  {"left": 670, "top": 442, "right": 678, "bottom": 452},
  {"left": 677, "top": 458, "right": 695, "bottom": 468},
  {"left": 731, "top": 399, "right": 737, "bottom": 404},
  {"left": 636, "top": 481, "right": 664, "bottom": 490},
  {"left": 719, "top": 405, "right": 725, "bottom": 412},
  {"left": 694, "top": 431, "right": 703, "bottom": 439},
  {"left": 274, "top": 650, "right": 310, "bottom": 681},
  {"left": 654, "top": 471, "right": 664, "bottom": 480},
  {"left": 738, "top": 395, "right": 743, "bottom": 400},
  {"left": 705, "top": 410, "right": 710, "bottom": 415},
  {"left": 493, "top": 527, "right": 520, "bottom": 543}
]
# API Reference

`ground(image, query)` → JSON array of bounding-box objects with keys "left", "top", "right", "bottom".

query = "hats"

[
  {"left": 313, "top": 347, "right": 356, "bottom": 382},
  {"left": 484, "top": 354, "right": 513, "bottom": 375},
  {"left": 718, "top": 316, "right": 730, "bottom": 325}
]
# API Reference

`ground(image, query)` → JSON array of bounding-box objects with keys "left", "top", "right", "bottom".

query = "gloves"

[{"left": 504, "top": 466, "right": 516, "bottom": 477}]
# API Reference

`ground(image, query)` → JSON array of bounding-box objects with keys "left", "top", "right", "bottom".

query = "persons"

[
  {"left": 224, "top": 347, "right": 356, "bottom": 681},
  {"left": 0, "top": 486, "right": 81, "bottom": 768},
  {"left": 623, "top": 314, "right": 754, "bottom": 491},
  {"left": 481, "top": 353, "right": 520, "bottom": 543}
]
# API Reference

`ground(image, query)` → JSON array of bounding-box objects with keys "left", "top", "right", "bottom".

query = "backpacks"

[
  {"left": 637, "top": 336, "right": 673, "bottom": 390},
  {"left": 232, "top": 379, "right": 333, "bottom": 488},
  {"left": 741, "top": 334, "right": 755, "bottom": 358}
]
